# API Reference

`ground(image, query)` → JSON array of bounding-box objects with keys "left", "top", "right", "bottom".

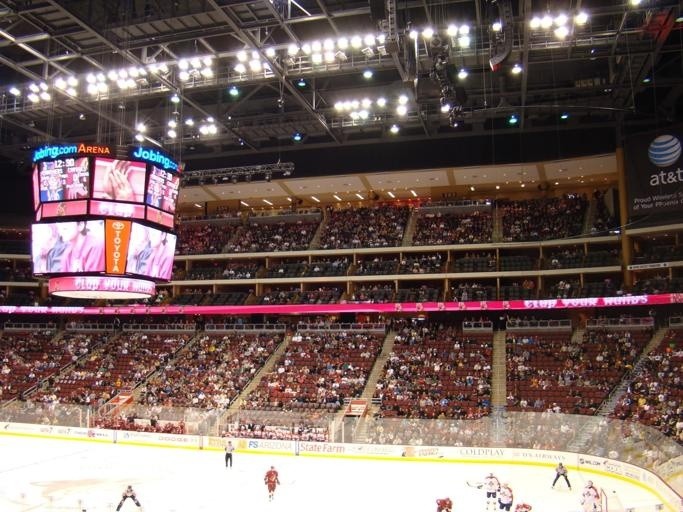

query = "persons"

[
  {"left": 579, "top": 479, "right": 601, "bottom": 511},
  {"left": 496, "top": 480, "right": 514, "bottom": 512},
  {"left": 511, "top": 502, "right": 531, "bottom": 512},
  {"left": 115, "top": 483, "right": 145, "bottom": 512},
  {"left": 435, "top": 495, "right": 452, "bottom": 512},
  {"left": 475, "top": 470, "right": 500, "bottom": 510},
  {"left": 3, "top": 161, "right": 683, "bottom": 471},
  {"left": 264, "top": 464, "right": 280, "bottom": 501}
]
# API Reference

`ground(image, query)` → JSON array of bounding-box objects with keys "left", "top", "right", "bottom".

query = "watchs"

[{"left": 550, "top": 461, "right": 572, "bottom": 492}]
